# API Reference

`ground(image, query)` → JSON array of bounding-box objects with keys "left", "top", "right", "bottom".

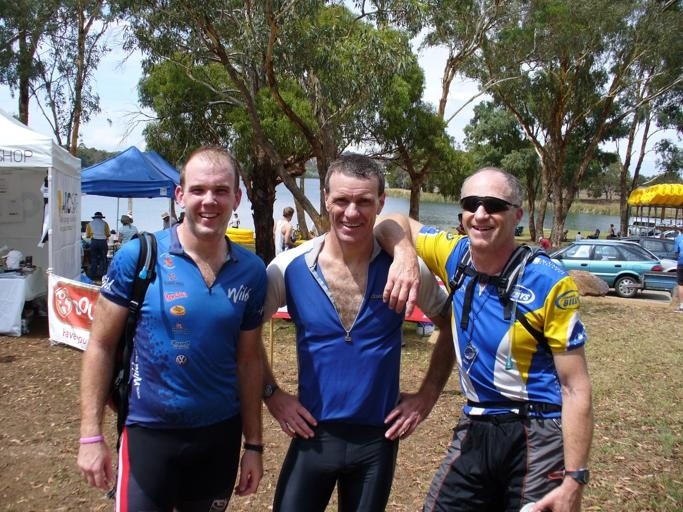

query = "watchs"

[
  {"left": 558, "top": 464, "right": 592, "bottom": 485},
  {"left": 260, "top": 381, "right": 279, "bottom": 400}
]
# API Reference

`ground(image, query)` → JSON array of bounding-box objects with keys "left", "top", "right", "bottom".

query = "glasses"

[{"left": 460, "top": 195, "right": 520, "bottom": 214}]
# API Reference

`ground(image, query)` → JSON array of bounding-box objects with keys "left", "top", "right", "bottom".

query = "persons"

[
  {"left": 258, "top": 152, "right": 456, "bottom": 510},
  {"left": 230, "top": 213, "right": 241, "bottom": 229},
  {"left": 68, "top": 146, "right": 269, "bottom": 510},
  {"left": 587, "top": 230, "right": 599, "bottom": 239},
  {"left": 538, "top": 236, "right": 551, "bottom": 250},
  {"left": 672, "top": 225, "right": 683, "bottom": 313},
  {"left": 610, "top": 224, "right": 615, "bottom": 237},
  {"left": 117, "top": 214, "right": 138, "bottom": 245},
  {"left": 85, "top": 212, "right": 111, "bottom": 279},
  {"left": 274, "top": 206, "right": 294, "bottom": 255},
  {"left": 576, "top": 232, "right": 581, "bottom": 240},
  {"left": 562, "top": 230, "right": 568, "bottom": 240},
  {"left": 370, "top": 164, "right": 599, "bottom": 511},
  {"left": 162, "top": 211, "right": 177, "bottom": 230},
  {"left": 457, "top": 213, "right": 467, "bottom": 235}
]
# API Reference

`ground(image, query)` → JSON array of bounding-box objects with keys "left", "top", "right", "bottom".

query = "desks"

[{"left": 0, "top": 265, "right": 41, "bottom": 337}]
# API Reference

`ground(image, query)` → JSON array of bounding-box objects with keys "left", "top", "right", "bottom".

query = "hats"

[
  {"left": 161, "top": 212, "right": 170, "bottom": 219},
  {"left": 92, "top": 212, "right": 105, "bottom": 218}
]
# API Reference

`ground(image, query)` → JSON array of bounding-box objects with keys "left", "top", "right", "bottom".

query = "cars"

[
  {"left": 542, "top": 238, "right": 660, "bottom": 298},
  {"left": 570, "top": 237, "right": 678, "bottom": 274},
  {"left": 628, "top": 218, "right": 656, "bottom": 235}
]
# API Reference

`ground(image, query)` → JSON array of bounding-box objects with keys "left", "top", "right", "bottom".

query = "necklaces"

[{"left": 461, "top": 284, "right": 496, "bottom": 362}]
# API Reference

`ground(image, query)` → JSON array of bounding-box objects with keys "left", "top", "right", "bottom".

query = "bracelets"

[
  {"left": 241, "top": 441, "right": 265, "bottom": 454},
  {"left": 77, "top": 433, "right": 104, "bottom": 445}
]
